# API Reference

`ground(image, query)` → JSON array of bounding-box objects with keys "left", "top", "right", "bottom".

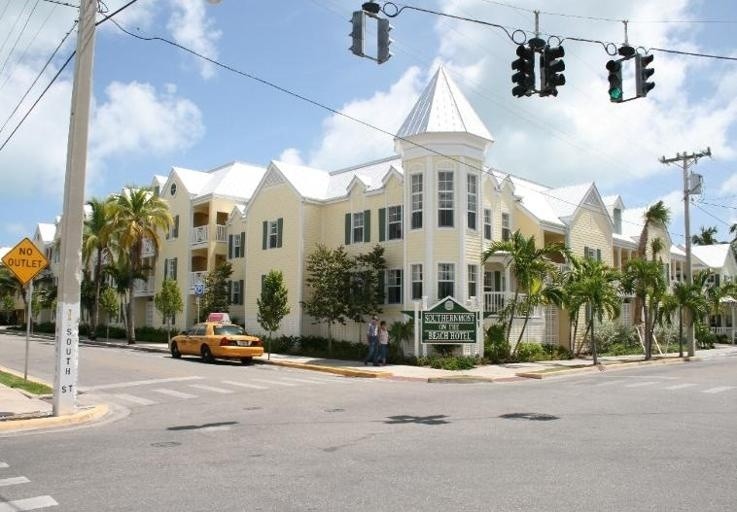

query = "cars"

[{"left": 166, "top": 312, "right": 264, "bottom": 366}]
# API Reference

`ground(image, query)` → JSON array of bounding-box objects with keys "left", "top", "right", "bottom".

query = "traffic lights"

[
  {"left": 542, "top": 45, "right": 564, "bottom": 96},
  {"left": 512, "top": 42, "right": 533, "bottom": 98},
  {"left": 642, "top": 54, "right": 653, "bottom": 96},
  {"left": 605, "top": 60, "right": 620, "bottom": 102},
  {"left": 348, "top": 10, "right": 364, "bottom": 56},
  {"left": 378, "top": 18, "right": 395, "bottom": 63}
]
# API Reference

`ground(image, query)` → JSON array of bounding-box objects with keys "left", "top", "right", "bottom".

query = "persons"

[
  {"left": 377, "top": 321, "right": 388, "bottom": 366},
  {"left": 364, "top": 316, "right": 380, "bottom": 366}
]
